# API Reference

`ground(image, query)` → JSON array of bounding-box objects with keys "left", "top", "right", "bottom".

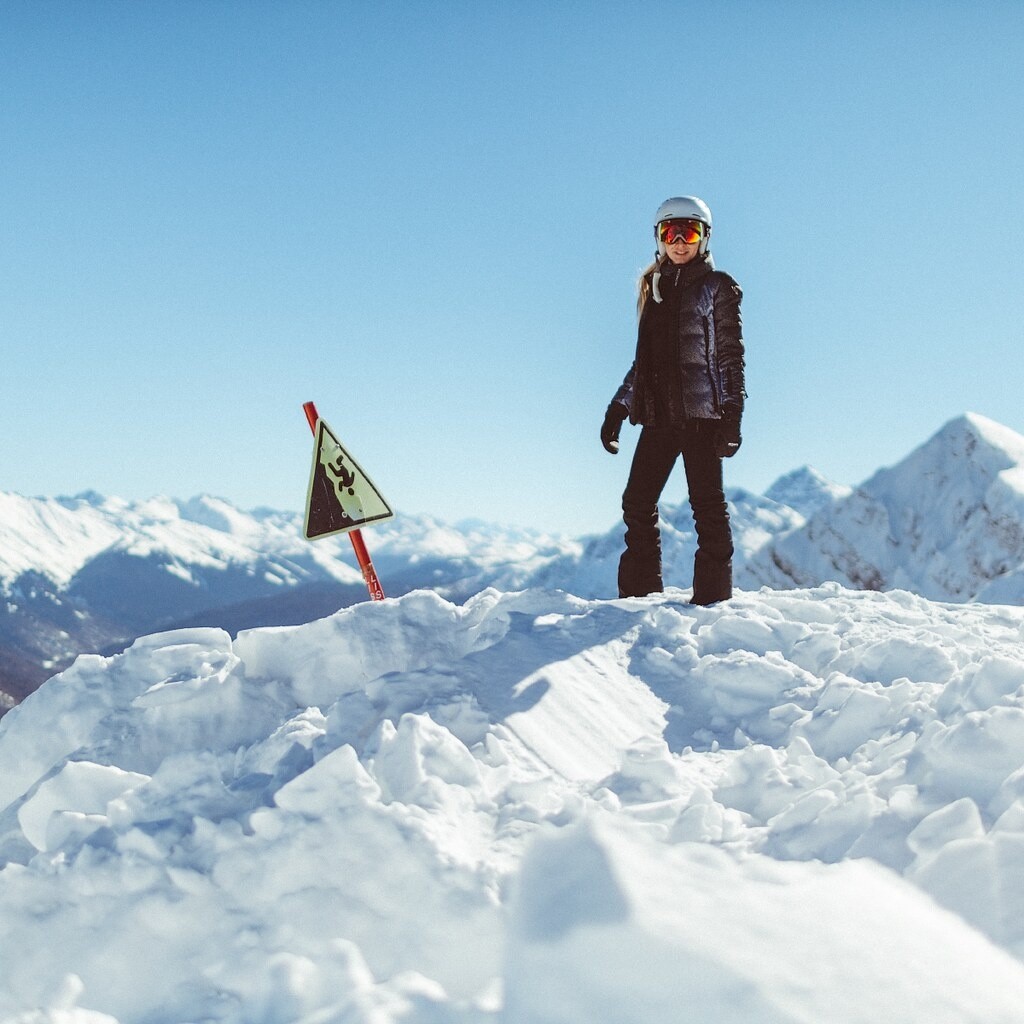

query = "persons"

[{"left": 601, "top": 196, "right": 748, "bottom": 606}]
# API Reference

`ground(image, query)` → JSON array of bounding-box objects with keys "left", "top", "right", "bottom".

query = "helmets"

[{"left": 655, "top": 195, "right": 712, "bottom": 254}]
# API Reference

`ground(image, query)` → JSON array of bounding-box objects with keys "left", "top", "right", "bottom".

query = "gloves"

[
  {"left": 716, "top": 404, "right": 742, "bottom": 457},
  {"left": 601, "top": 403, "right": 629, "bottom": 453}
]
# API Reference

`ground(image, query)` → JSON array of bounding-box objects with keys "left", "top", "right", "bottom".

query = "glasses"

[{"left": 657, "top": 220, "right": 706, "bottom": 244}]
